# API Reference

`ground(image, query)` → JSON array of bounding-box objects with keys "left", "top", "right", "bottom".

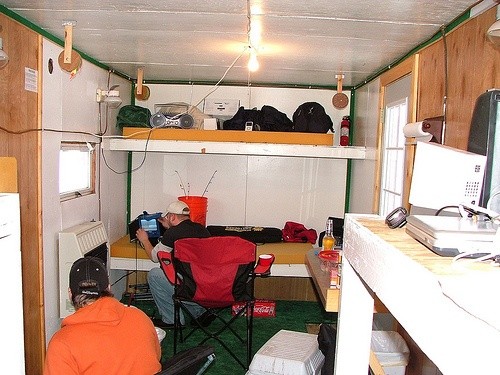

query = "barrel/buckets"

[{"left": 178, "top": 196, "right": 207, "bottom": 227}]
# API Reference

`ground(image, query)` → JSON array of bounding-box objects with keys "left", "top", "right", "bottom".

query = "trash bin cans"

[{"left": 370, "top": 329, "right": 411, "bottom": 375}]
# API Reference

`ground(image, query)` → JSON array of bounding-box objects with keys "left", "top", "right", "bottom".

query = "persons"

[
  {"left": 134, "top": 199, "right": 219, "bottom": 329},
  {"left": 43, "top": 255, "right": 163, "bottom": 375}
]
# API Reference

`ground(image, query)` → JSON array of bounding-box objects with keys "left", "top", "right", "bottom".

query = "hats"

[
  {"left": 161, "top": 200, "right": 191, "bottom": 218},
  {"left": 69, "top": 257, "right": 109, "bottom": 292}
]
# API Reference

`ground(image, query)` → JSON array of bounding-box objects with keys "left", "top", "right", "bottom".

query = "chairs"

[
  {"left": 157, "top": 236, "right": 275, "bottom": 370},
  {"left": 153, "top": 347, "right": 217, "bottom": 375}
]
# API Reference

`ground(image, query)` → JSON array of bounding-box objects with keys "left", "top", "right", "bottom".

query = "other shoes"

[
  {"left": 158, "top": 320, "right": 184, "bottom": 330},
  {"left": 191, "top": 310, "right": 216, "bottom": 327}
]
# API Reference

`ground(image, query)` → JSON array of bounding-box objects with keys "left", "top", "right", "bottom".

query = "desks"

[
  {"left": 109, "top": 235, "right": 313, "bottom": 277},
  {"left": 306, "top": 248, "right": 390, "bottom": 314}
]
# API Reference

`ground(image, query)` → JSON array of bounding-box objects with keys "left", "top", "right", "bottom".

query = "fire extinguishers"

[{"left": 340, "top": 116, "right": 352, "bottom": 145}]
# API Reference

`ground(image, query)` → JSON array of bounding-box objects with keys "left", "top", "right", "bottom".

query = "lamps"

[
  {"left": 97, "top": 90, "right": 122, "bottom": 110},
  {"left": 486, "top": 4, "right": 500, "bottom": 37},
  {"left": 0, "top": 38, "right": 9, "bottom": 69}
]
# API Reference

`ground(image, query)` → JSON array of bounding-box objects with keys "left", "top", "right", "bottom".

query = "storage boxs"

[
  {"left": 245, "top": 327, "right": 326, "bottom": 375},
  {"left": 204, "top": 98, "right": 239, "bottom": 115},
  {"left": 232, "top": 297, "right": 276, "bottom": 318},
  {"left": 0, "top": 156, "right": 20, "bottom": 237}
]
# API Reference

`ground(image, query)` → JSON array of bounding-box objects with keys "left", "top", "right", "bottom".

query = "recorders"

[{"left": 150, "top": 113, "right": 194, "bottom": 129}]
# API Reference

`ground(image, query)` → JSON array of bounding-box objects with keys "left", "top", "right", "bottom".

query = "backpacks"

[{"left": 293, "top": 102, "right": 333, "bottom": 134}]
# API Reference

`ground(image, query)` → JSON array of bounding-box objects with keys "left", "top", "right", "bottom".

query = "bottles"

[{"left": 321, "top": 220, "right": 335, "bottom": 251}]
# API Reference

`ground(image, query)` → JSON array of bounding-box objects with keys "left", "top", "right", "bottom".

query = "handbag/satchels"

[
  {"left": 222, "top": 106, "right": 294, "bottom": 132},
  {"left": 116, "top": 105, "right": 150, "bottom": 128},
  {"left": 282, "top": 221, "right": 317, "bottom": 244}
]
